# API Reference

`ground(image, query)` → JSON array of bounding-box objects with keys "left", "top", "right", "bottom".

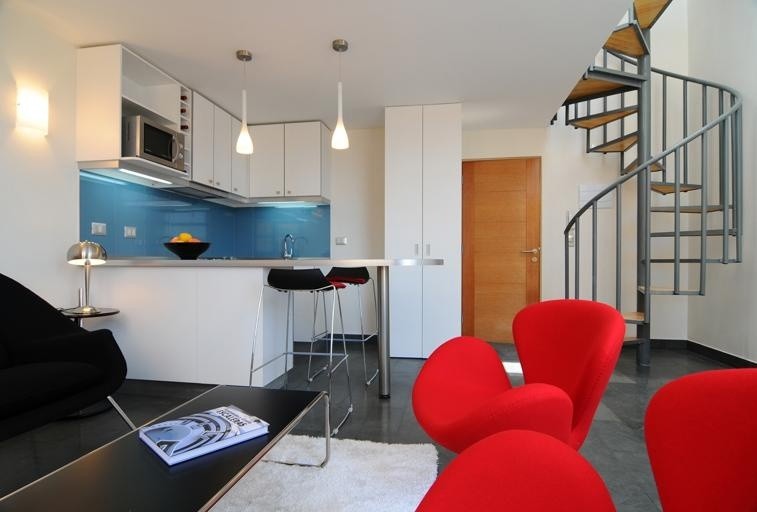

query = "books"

[{"left": 139, "top": 403, "right": 270, "bottom": 464}]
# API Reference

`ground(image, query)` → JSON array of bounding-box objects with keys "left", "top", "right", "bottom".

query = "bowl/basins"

[{"left": 163, "top": 241, "right": 210, "bottom": 260}]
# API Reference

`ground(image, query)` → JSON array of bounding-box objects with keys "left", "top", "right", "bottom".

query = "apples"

[{"left": 171, "top": 237, "right": 179, "bottom": 242}]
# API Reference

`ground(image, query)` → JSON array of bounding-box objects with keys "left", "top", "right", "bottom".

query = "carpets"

[{"left": 208, "top": 432, "right": 440, "bottom": 512}]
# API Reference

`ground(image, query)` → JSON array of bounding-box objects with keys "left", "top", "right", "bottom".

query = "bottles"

[{"left": 180, "top": 95, "right": 188, "bottom": 130}]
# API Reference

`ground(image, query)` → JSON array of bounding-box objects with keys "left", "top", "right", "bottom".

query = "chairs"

[
  {"left": 408, "top": 298, "right": 626, "bottom": 455},
  {"left": 413, "top": 425, "right": 618, "bottom": 512},
  {"left": 642, "top": 365, "right": 756, "bottom": 512}
]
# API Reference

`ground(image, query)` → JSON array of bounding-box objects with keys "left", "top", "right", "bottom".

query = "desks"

[
  {"left": 60, "top": 305, "right": 120, "bottom": 331},
  {"left": 82, "top": 255, "right": 445, "bottom": 401}
]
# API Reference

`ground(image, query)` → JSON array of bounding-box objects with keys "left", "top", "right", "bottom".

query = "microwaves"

[{"left": 121, "top": 115, "right": 185, "bottom": 173}]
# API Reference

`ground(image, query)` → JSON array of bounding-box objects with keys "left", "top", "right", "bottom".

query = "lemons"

[{"left": 180, "top": 233, "right": 191, "bottom": 242}]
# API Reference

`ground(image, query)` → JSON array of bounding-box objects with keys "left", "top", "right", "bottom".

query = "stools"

[
  {"left": 305, "top": 265, "right": 380, "bottom": 386},
  {"left": 247, "top": 267, "right": 356, "bottom": 437}
]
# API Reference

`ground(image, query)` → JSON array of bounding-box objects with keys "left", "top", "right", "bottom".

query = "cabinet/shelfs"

[
  {"left": 76, "top": 43, "right": 190, "bottom": 189},
  {"left": 246, "top": 119, "right": 330, "bottom": 204},
  {"left": 385, "top": 100, "right": 463, "bottom": 360},
  {"left": 163, "top": 90, "right": 229, "bottom": 200},
  {"left": 205, "top": 113, "right": 246, "bottom": 207}
]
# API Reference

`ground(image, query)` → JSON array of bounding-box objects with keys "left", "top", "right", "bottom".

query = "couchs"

[{"left": 0, "top": 270, "right": 128, "bottom": 446}]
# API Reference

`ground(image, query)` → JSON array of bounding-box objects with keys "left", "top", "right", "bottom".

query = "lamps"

[
  {"left": 64, "top": 239, "right": 108, "bottom": 315},
  {"left": 234, "top": 50, "right": 254, "bottom": 155},
  {"left": 330, "top": 37, "right": 351, "bottom": 149}
]
